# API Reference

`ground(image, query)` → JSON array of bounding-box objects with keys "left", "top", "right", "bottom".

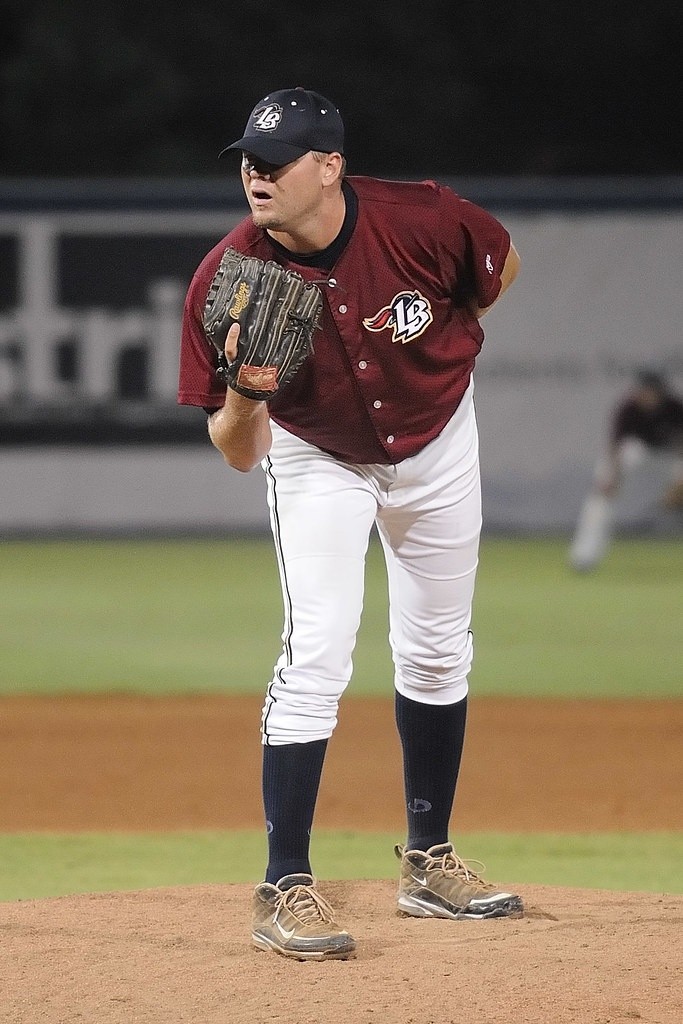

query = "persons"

[
  {"left": 177, "top": 88, "right": 522, "bottom": 961},
  {"left": 568, "top": 370, "right": 683, "bottom": 574}
]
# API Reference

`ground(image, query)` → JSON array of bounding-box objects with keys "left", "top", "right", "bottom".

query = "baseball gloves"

[{"left": 202, "top": 246, "right": 323, "bottom": 400}]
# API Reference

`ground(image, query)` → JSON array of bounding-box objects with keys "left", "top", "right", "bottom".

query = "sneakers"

[
  {"left": 250, "top": 873, "right": 356, "bottom": 960},
  {"left": 394, "top": 843, "right": 523, "bottom": 919}
]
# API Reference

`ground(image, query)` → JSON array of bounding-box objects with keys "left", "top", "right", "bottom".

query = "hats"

[{"left": 219, "top": 87, "right": 346, "bottom": 165}]
